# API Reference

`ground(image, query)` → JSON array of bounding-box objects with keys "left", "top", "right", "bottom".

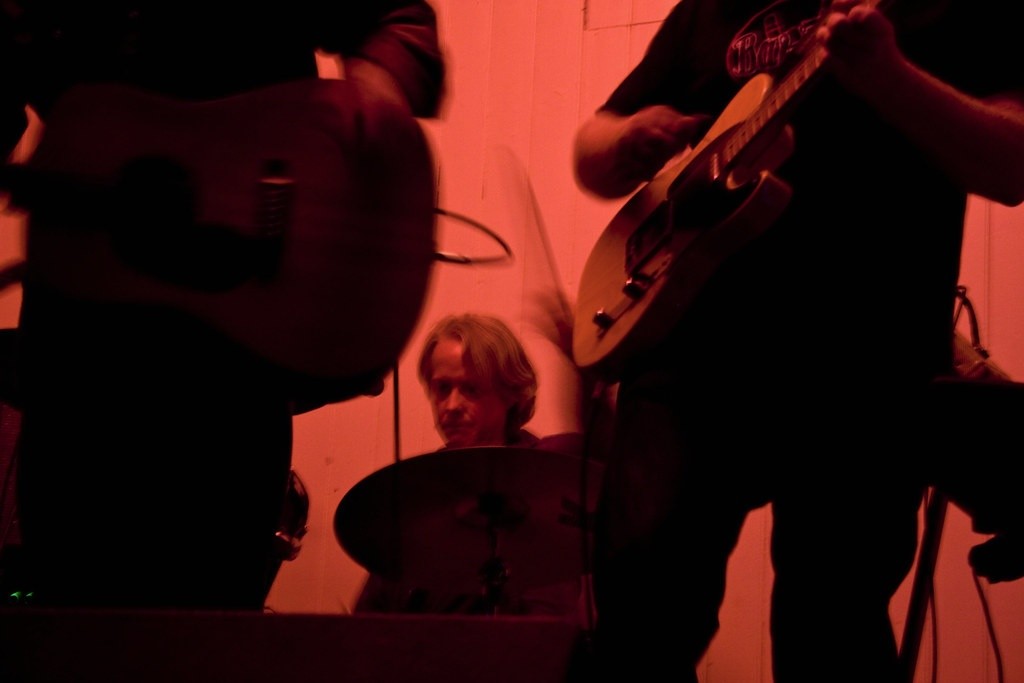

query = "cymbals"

[{"left": 333, "top": 446, "right": 601, "bottom": 591}]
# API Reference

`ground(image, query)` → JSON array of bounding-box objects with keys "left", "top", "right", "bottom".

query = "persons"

[
  {"left": 567, "top": 0, "right": 1024, "bottom": 683},
  {"left": 352, "top": 293, "right": 613, "bottom": 619},
  {"left": 0, "top": 0, "right": 446, "bottom": 610}
]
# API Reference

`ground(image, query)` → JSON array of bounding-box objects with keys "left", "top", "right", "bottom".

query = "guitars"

[
  {"left": 570, "top": 0, "right": 887, "bottom": 382},
  {"left": 0, "top": 78, "right": 438, "bottom": 388}
]
